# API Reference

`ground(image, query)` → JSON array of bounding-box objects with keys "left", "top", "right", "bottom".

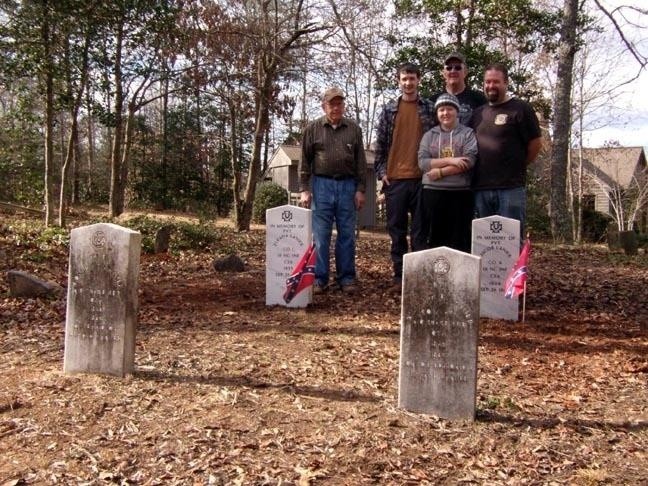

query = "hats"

[
  {"left": 434, "top": 94, "right": 461, "bottom": 113},
  {"left": 443, "top": 51, "right": 465, "bottom": 65},
  {"left": 321, "top": 88, "right": 346, "bottom": 102}
]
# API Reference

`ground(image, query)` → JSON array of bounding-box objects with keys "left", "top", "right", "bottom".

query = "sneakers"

[
  {"left": 388, "top": 282, "right": 401, "bottom": 295},
  {"left": 314, "top": 285, "right": 329, "bottom": 295},
  {"left": 342, "top": 285, "right": 355, "bottom": 294}
]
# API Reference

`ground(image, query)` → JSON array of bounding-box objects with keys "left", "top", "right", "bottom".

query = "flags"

[
  {"left": 281, "top": 240, "right": 317, "bottom": 305},
  {"left": 502, "top": 231, "right": 530, "bottom": 301}
]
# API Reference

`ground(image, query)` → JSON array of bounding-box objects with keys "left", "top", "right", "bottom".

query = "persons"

[
  {"left": 467, "top": 61, "right": 542, "bottom": 255},
  {"left": 415, "top": 91, "right": 478, "bottom": 252},
  {"left": 297, "top": 86, "right": 368, "bottom": 294},
  {"left": 427, "top": 50, "right": 486, "bottom": 128},
  {"left": 373, "top": 62, "right": 440, "bottom": 294}
]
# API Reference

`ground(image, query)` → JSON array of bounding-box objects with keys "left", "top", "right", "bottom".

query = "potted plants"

[{"left": 580, "top": 153, "right": 647, "bottom": 254}]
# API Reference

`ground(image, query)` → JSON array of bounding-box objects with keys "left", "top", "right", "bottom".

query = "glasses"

[{"left": 446, "top": 65, "right": 463, "bottom": 71}]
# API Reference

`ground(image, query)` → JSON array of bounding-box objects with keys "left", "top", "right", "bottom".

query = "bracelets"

[{"left": 436, "top": 166, "right": 443, "bottom": 179}]
